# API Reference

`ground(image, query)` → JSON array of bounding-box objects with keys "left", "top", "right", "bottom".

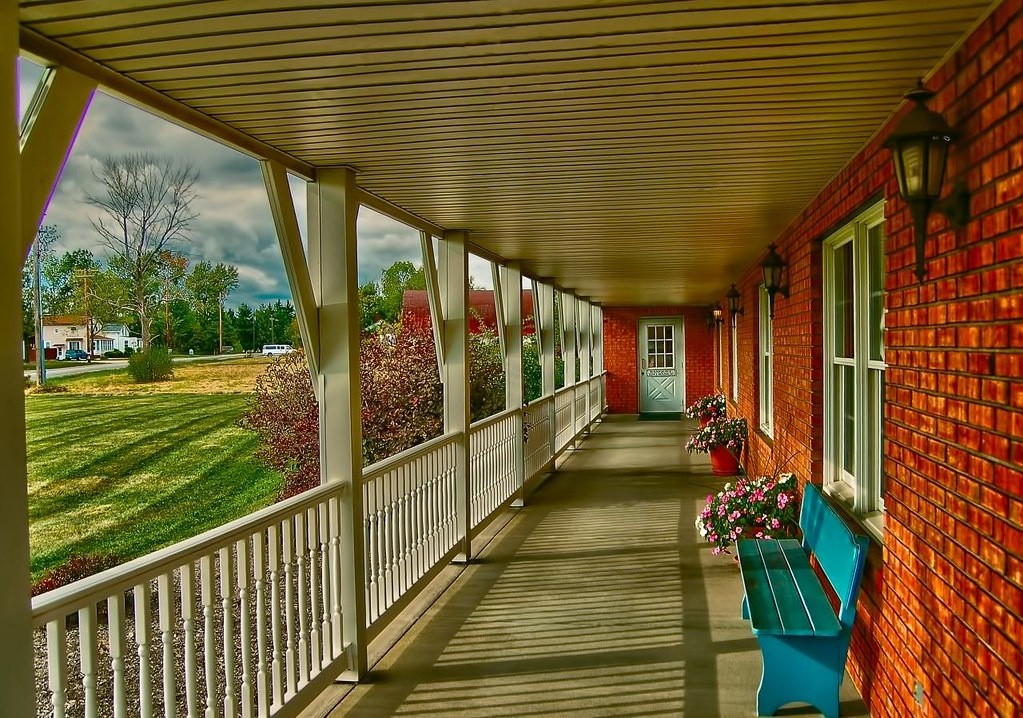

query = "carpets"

[{"left": 637, "top": 412, "right": 681, "bottom": 421}]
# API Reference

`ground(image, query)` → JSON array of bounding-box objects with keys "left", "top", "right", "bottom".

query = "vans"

[{"left": 262, "top": 344, "right": 298, "bottom": 358}]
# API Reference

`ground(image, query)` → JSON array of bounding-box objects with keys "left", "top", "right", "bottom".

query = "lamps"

[
  {"left": 880, "top": 76, "right": 969, "bottom": 284},
  {"left": 759, "top": 242, "right": 789, "bottom": 319},
  {"left": 707, "top": 302, "right": 724, "bottom": 334},
  {"left": 726, "top": 281, "right": 744, "bottom": 330}
]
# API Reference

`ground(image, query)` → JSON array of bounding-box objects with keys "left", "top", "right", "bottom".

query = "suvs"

[{"left": 65, "top": 350, "right": 88, "bottom": 360}]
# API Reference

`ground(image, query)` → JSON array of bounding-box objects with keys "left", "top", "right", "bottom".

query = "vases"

[
  {"left": 711, "top": 445, "right": 741, "bottom": 476},
  {"left": 745, "top": 527, "right": 765, "bottom": 539},
  {"left": 733, "top": 554, "right": 739, "bottom": 566},
  {"left": 700, "top": 415, "right": 717, "bottom": 425}
]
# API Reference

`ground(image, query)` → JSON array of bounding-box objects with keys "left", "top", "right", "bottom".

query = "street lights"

[{"left": 269, "top": 315, "right": 279, "bottom": 345}]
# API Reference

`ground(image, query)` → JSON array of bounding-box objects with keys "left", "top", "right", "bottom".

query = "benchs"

[{"left": 736, "top": 481, "right": 869, "bottom": 718}]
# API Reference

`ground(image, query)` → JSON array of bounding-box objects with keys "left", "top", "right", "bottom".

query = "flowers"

[
  {"left": 689, "top": 421, "right": 800, "bottom": 556},
  {"left": 684, "top": 416, "right": 748, "bottom": 455},
  {"left": 686, "top": 394, "right": 725, "bottom": 419}
]
